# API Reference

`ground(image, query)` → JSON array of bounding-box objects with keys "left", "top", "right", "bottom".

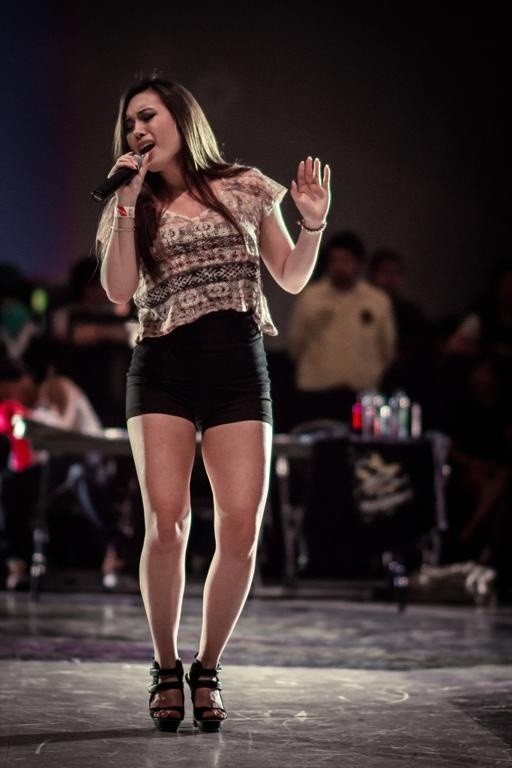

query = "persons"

[
  {"left": 95, "top": 75, "right": 331, "bottom": 732},
  {"left": 2, "top": 229, "right": 511, "bottom": 602}
]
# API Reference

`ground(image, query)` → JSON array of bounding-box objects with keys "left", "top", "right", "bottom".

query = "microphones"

[{"left": 91, "top": 154, "right": 142, "bottom": 203}]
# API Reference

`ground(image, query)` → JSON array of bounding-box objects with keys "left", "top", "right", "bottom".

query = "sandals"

[
  {"left": 148, "top": 655, "right": 185, "bottom": 733},
  {"left": 186, "top": 652, "right": 228, "bottom": 734}
]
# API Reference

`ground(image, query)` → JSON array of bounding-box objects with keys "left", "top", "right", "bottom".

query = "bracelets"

[
  {"left": 297, "top": 219, "right": 327, "bottom": 233},
  {"left": 110, "top": 225, "right": 136, "bottom": 234},
  {"left": 112, "top": 206, "right": 135, "bottom": 217}
]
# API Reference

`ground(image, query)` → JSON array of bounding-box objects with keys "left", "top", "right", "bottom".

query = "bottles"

[{"left": 351, "top": 387, "right": 422, "bottom": 439}]
